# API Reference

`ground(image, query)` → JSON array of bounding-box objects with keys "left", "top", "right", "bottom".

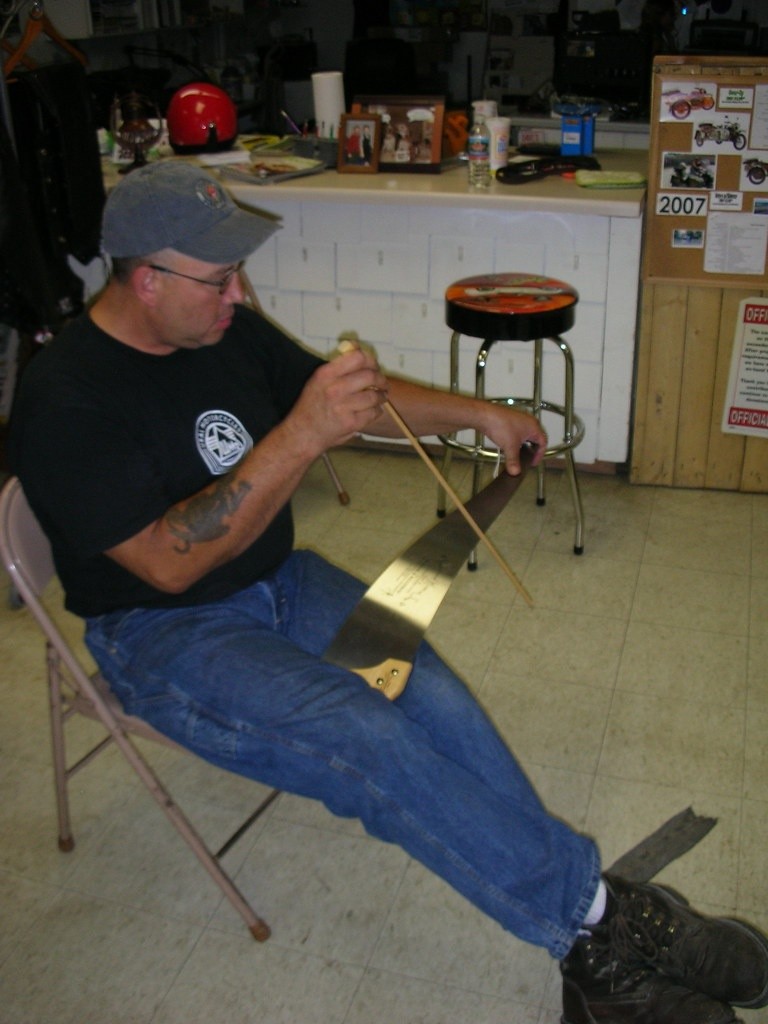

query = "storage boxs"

[{"left": 292, "top": 135, "right": 341, "bottom": 169}]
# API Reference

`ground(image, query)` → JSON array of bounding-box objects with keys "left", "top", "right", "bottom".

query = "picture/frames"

[
  {"left": 344, "top": 94, "right": 444, "bottom": 174},
  {"left": 338, "top": 115, "right": 381, "bottom": 173}
]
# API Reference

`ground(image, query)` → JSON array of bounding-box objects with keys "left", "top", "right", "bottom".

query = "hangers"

[{"left": 0, "top": 0, "right": 88, "bottom": 87}]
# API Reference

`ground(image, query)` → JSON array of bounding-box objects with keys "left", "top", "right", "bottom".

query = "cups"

[
  {"left": 312, "top": 72, "right": 345, "bottom": 139},
  {"left": 472, "top": 101, "right": 498, "bottom": 125},
  {"left": 484, "top": 117, "right": 512, "bottom": 178}
]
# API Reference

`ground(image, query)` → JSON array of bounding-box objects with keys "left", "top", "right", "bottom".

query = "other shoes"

[{"left": 557, "top": 873, "right": 768, "bottom": 1024}]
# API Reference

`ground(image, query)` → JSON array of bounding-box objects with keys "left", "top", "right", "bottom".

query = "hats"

[{"left": 100, "top": 159, "right": 283, "bottom": 263}]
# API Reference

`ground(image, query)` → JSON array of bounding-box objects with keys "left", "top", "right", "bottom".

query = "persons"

[
  {"left": 5, "top": 157, "right": 768, "bottom": 1024},
  {"left": 345, "top": 124, "right": 417, "bottom": 163}
]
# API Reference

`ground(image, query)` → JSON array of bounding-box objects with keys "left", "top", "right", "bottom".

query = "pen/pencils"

[{"left": 280, "top": 109, "right": 333, "bottom": 141}]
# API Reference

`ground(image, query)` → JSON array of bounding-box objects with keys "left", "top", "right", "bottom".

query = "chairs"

[{"left": 0, "top": 475, "right": 282, "bottom": 942}]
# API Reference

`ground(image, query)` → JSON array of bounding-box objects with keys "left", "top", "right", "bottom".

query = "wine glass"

[{"left": 108, "top": 95, "right": 165, "bottom": 174}]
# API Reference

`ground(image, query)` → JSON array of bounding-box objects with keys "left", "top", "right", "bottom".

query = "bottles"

[{"left": 468, "top": 114, "right": 491, "bottom": 188}]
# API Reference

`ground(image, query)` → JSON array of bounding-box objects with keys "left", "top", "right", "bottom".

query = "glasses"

[{"left": 138, "top": 258, "right": 245, "bottom": 294}]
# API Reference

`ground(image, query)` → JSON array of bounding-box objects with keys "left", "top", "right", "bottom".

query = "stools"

[{"left": 436, "top": 272, "right": 588, "bottom": 572}]
